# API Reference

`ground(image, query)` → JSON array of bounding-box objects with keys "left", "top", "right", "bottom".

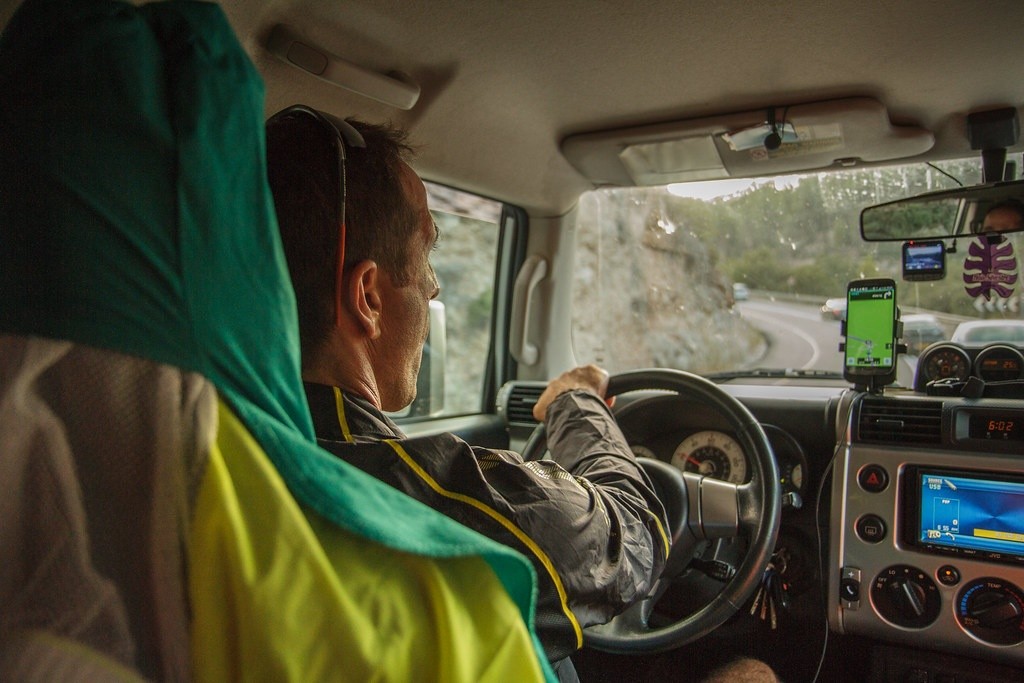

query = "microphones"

[{"left": 764, "top": 107, "right": 782, "bottom": 151}]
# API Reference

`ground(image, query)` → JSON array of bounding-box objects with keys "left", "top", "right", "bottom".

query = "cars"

[
  {"left": 953, "top": 318, "right": 1022, "bottom": 348},
  {"left": 897, "top": 313, "right": 946, "bottom": 354},
  {"left": 819, "top": 295, "right": 850, "bottom": 318},
  {"left": 732, "top": 283, "right": 751, "bottom": 300}
]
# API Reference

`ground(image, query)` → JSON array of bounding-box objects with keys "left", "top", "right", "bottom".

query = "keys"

[{"left": 751, "top": 552, "right": 790, "bottom": 630}]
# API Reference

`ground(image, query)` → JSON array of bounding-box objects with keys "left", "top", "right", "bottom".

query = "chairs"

[{"left": 0, "top": 0, "right": 584, "bottom": 683}]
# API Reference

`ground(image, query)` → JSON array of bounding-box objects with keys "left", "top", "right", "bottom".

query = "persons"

[{"left": 266, "top": 103, "right": 777, "bottom": 683}]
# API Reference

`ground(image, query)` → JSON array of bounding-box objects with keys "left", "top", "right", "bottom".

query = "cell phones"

[{"left": 843, "top": 278, "right": 899, "bottom": 375}]
